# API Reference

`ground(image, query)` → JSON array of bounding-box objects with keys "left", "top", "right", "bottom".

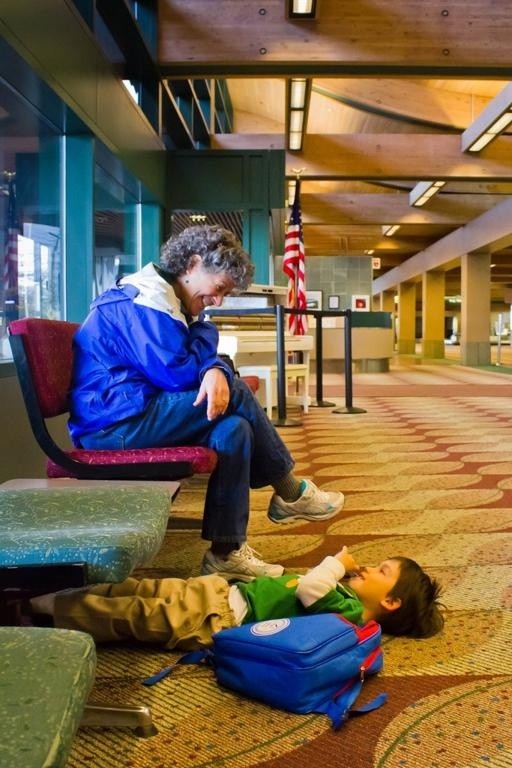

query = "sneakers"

[
  {"left": 201, "top": 547, "right": 285, "bottom": 583},
  {"left": 267, "top": 478, "right": 348, "bottom": 526}
]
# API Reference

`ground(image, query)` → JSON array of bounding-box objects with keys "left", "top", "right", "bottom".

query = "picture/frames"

[
  {"left": 305, "top": 289, "right": 323, "bottom": 311},
  {"left": 328, "top": 295, "right": 340, "bottom": 309},
  {"left": 352, "top": 295, "right": 370, "bottom": 313}
]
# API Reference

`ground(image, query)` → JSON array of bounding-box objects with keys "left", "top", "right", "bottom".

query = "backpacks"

[{"left": 208, "top": 612, "right": 389, "bottom": 717}]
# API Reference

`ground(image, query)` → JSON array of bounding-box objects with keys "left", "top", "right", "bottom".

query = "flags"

[
  {"left": 282, "top": 179, "right": 309, "bottom": 364},
  {"left": 0, "top": 177, "right": 26, "bottom": 291}
]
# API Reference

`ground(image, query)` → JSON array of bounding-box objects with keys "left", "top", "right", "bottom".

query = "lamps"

[
  {"left": 285, "top": 78, "right": 311, "bottom": 153},
  {"left": 285, "top": 180, "right": 297, "bottom": 209},
  {"left": 409, "top": 181, "right": 446, "bottom": 208},
  {"left": 461, "top": 81, "right": 512, "bottom": 155},
  {"left": 364, "top": 250, "right": 375, "bottom": 256},
  {"left": 286, "top": 0, "right": 321, "bottom": 21},
  {"left": 381, "top": 225, "right": 400, "bottom": 238}
]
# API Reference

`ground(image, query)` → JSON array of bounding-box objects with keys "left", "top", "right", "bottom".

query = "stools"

[
  {"left": 0, "top": 477, "right": 181, "bottom": 737},
  {"left": 239, "top": 363, "right": 310, "bottom": 424},
  {"left": 0, "top": 626, "right": 97, "bottom": 768}
]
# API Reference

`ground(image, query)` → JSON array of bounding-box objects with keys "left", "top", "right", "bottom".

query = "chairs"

[{"left": 5, "top": 316, "right": 216, "bottom": 528}]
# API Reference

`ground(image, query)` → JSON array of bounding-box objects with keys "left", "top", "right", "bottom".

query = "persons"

[
  {"left": 17, "top": 544, "right": 448, "bottom": 655},
  {"left": 68, "top": 224, "right": 347, "bottom": 583}
]
